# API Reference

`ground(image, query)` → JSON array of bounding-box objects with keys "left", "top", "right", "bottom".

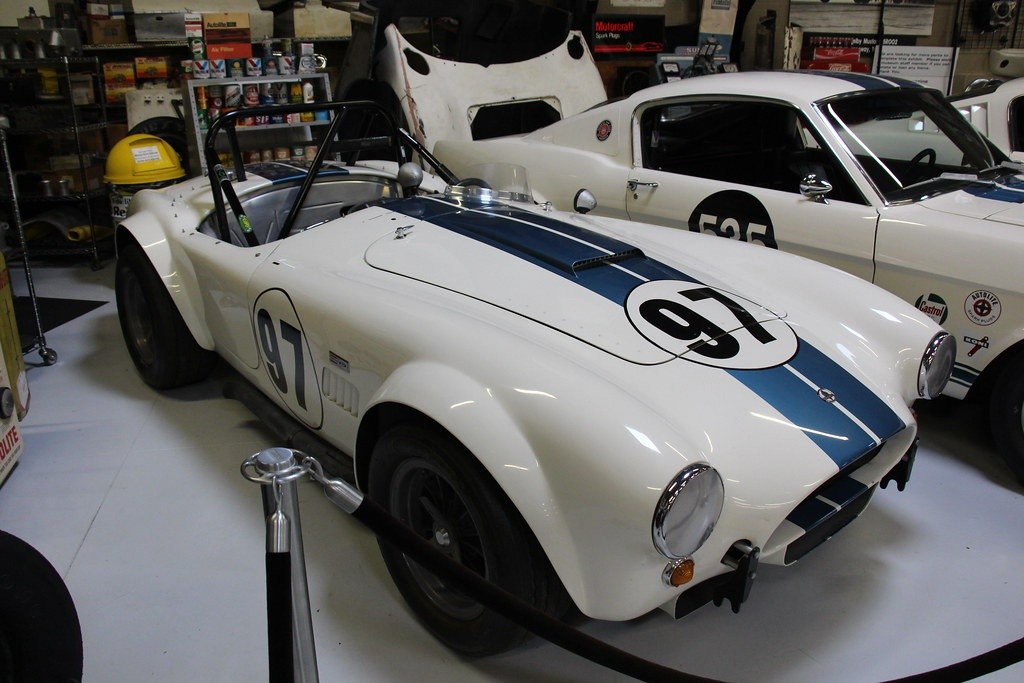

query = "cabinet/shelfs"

[
  {"left": 0, "top": 56, "right": 112, "bottom": 275},
  {"left": 185, "top": 72, "right": 342, "bottom": 174}
]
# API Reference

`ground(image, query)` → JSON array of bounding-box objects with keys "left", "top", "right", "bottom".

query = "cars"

[
  {"left": 111, "top": 98, "right": 960, "bottom": 659},
  {"left": 379, "top": 26, "right": 1024, "bottom": 483},
  {"left": 834, "top": 75, "right": 1024, "bottom": 166}
]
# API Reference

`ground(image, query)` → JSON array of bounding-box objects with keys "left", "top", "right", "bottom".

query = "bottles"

[
  {"left": 264, "top": 43, "right": 272, "bottom": 55},
  {"left": 303, "top": 80, "right": 315, "bottom": 104},
  {"left": 262, "top": 35, "right": 272, "bottom": 47}
]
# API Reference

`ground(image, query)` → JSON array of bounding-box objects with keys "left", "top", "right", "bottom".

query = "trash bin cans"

[{"left": 103, "top": 133, "right": 187, "bottom": 231}]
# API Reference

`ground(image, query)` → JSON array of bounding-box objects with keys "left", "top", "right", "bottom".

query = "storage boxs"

[
  {"left": 194, "top": 10, "right": 253, "bottom": 59},
  {"left": 40, "top": 0, "right": 172, "bottom": 194}
]
